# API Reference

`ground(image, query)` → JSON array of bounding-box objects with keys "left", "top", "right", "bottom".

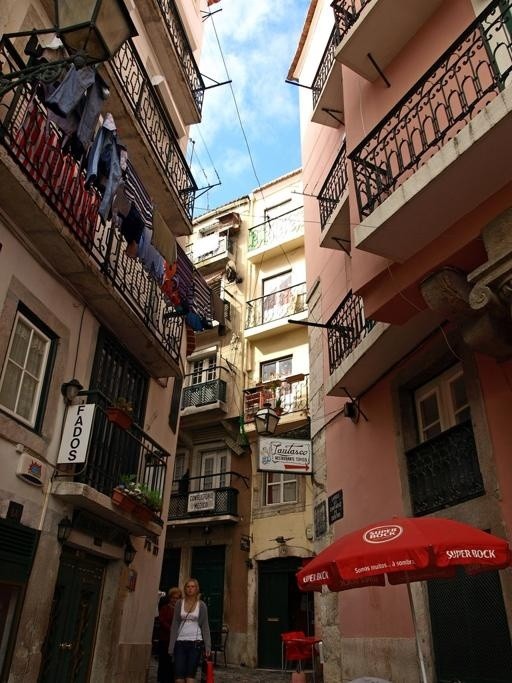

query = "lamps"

[
  {"left": 124, "top": 542, "right": 138, "bottom": 567},
  {"left": 57, "top": 515, "right": 72, "bottom": 546},
  {"left": 61, "top": 379, "right": 83, "bottom": 406},
  {"left": 254, "top": 403, "right": 280, "bottom": 437},
  {"left": 1, "top": 0, "right": 139, "bottom": 103}
]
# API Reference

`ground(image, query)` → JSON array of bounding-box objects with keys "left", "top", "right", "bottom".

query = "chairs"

[{"left": 209, "top": 624, "right": 229, "bottom": 669}]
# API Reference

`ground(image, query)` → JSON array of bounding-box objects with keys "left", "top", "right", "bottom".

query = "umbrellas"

[{"left": 296, "top": 517, "right": 511, "bottom": 683}]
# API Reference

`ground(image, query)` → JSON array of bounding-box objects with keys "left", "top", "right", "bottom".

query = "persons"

[
  {"left": 157, "top": 588, "right": 182, "bottom": 682},
  {"left": 168, "top": 578, "right": 212, "bottom": 682}
]
